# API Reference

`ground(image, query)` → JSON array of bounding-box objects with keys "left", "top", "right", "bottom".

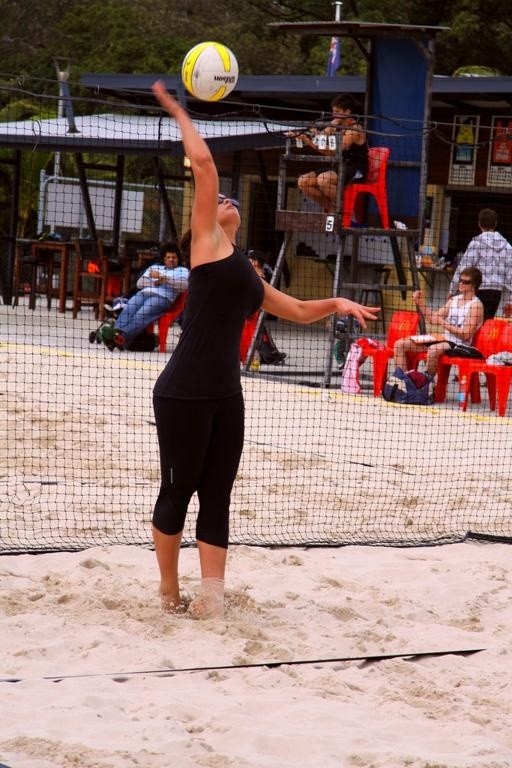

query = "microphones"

[{"left": 358, "top": 288, "right": 386, "bottom": 335}]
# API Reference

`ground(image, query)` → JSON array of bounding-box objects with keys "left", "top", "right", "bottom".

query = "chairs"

[
  {"left": 102, "top": 326, "right": 127, "bottom": 347},
  {"left": 99, "top": 332, "right": 116, "bottom": 351}
]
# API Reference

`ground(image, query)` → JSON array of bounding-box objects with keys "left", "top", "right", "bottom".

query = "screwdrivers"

[{"left": 183, "top": 43, "right": 240, "bottom": 103}]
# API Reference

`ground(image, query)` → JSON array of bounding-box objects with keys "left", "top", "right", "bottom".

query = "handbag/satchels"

[{"left": 158, "top": 273, "right": 165, "bottom": 281}]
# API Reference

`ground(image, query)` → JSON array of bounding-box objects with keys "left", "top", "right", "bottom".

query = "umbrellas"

[{"left": 265, "top": 351, "right": 286, "bottom": 363}]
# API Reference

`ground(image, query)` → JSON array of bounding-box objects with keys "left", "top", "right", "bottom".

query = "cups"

[
  {"left": 459, "top": 278, "right": 473, "bottom": 284},
  {"left": 217, "top": 196, "right": 240, "bottom": 209}
]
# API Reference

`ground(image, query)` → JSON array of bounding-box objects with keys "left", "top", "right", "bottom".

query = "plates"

[
  {"left": 314, "top": 257, "right": 385, "bottom": 279},
  {"left": 409, "top": 265, "right": 456, "bottom": 290}
]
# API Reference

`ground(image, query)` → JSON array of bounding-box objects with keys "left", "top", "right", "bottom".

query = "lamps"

[
  {"left": 350, "top": 309, "right": 512, "bottom": 417},
  {"left": 341, "top": 146, "right": 391, "bottom": 231},
  {"left": 114, "top": 288, "right": 188, "bottom": 352},
  {"left": 14, "top": 238, "right": 160, "bottom": 322}
]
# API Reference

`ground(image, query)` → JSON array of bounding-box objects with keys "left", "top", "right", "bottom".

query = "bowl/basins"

[{"left": 102, "top": 244, "right": 191, "bottom": 351}]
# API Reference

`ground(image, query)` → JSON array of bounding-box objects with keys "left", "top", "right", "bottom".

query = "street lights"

[{"left": 383, "top": 366, "right": 435, "bottom": 404}]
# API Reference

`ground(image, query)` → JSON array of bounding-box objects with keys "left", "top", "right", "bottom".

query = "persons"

[
  {"left": 445, "top": 208, "right": 511, "bottom": 327},
  {"left": 394, "top": 267, "right": 483, "bottom": 385},
  {"left": 242, "top": 249, "right": 265, "bottom": 282},
  {"left": 150, "top": 69, "right": 385, "bottom": 621},
  {"left": 286, "top": 95, "right": 367, "bottom": 222}
]
